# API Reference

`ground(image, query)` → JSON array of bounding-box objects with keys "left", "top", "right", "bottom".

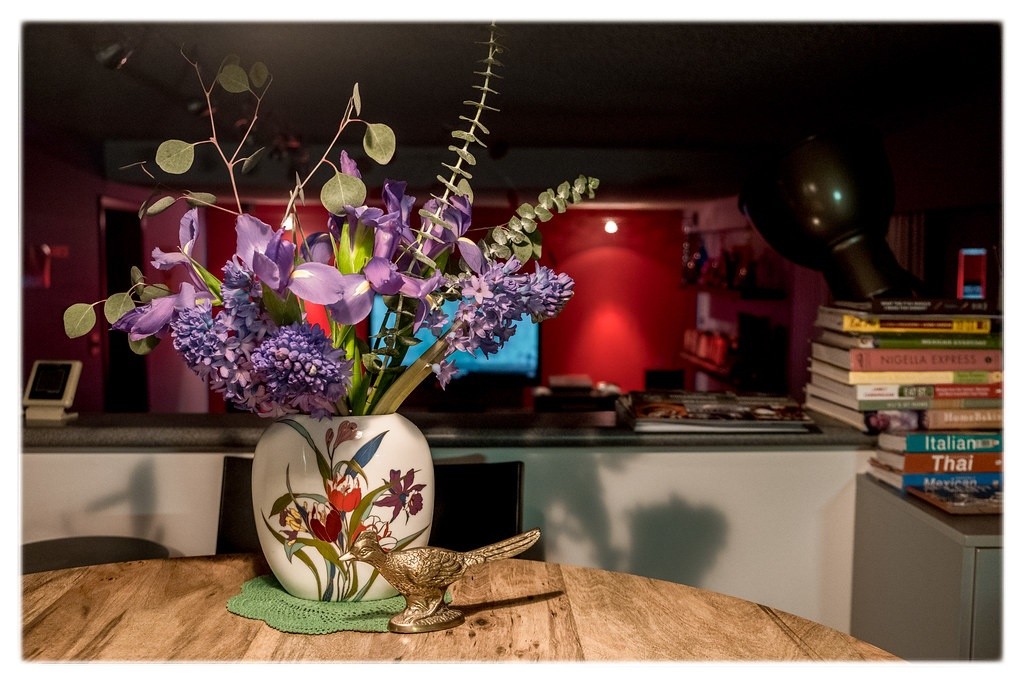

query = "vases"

[{"left": 251, "top": 409, "right": 436, "bottom": 600}]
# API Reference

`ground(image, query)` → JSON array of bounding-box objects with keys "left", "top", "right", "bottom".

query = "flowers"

[{"left": 61, "top": 22, "right": 603, "bottom": 421}]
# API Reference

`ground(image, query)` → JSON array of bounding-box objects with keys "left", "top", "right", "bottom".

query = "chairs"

[
  {"left": 215, "top": 456, "right": 522, "bottom": 551},
  {"left": 21, "top": 536, "right": 170, "bottom": 578}
]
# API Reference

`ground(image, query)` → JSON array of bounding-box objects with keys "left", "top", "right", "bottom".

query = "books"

[
  {"left": 616, "top": 390, "right": 814, "bottom": 433},
  {"left": 802, "top": 300, "right": 1003, "bottom": 515}
]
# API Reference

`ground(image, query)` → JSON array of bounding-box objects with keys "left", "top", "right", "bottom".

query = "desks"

[{"left": 20, "top": 553, "right": 918, "bottom": 663}]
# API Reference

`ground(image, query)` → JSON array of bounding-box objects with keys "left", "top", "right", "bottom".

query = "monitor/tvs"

[{"left": 366, "top": 291, "right": 543, "bottom": 391}]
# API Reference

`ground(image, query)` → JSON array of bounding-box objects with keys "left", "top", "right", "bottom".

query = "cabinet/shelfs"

[{"left": 844, "top": 472, "right": 1003, "bottom": 662}]
